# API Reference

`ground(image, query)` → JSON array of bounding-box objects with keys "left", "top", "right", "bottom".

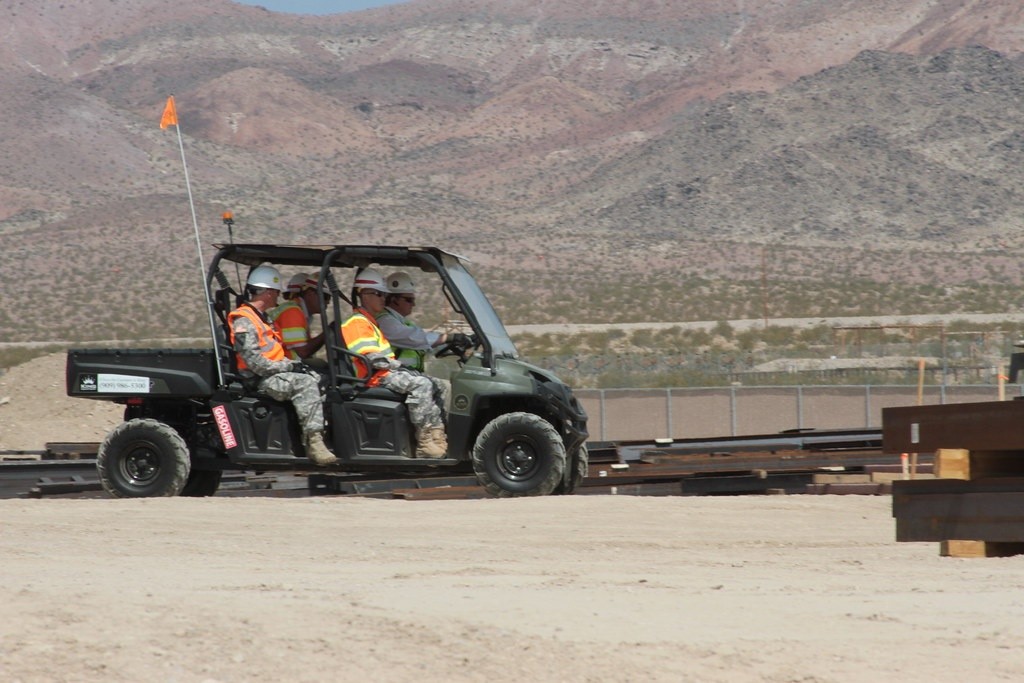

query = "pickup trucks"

[{"left": 65, "top": 240, "right": 590, "bottom": 500}]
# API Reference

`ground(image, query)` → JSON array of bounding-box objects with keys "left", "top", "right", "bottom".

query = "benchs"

[
  {"left": 227, "top": 380, "right": 288, "bottom": 409},
  {"left": 339, "top": 368, "right": 406, "bottom": 402}
]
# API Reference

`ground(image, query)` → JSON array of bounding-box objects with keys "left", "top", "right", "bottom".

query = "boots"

[
  {"left": 431, "top": 427, "right": 448, "bottom": 452},
  {"left": 414, "top": 424, "right": 447, "bottom": 459},
  {"left": 302, "top": 431, "right": 336, "bottom": 464}
]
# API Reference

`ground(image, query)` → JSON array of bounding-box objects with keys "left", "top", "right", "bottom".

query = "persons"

[
  {"left": 227, "top": 265, "right": 336, "bottom": 464},
  {"left": 267, "top": 271, "right": 336, "bottom": 386},
  {"left": 374, "top": 272, "right": 473, "bottom": 433},
  {"left": 342, "top": 268, "right": 448, "bottom": 459}
]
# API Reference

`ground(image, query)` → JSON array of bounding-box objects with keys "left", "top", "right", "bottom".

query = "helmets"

[
  {"left": 352, "top": 267, "right": 392, "bottom": 294},
  {"left": 283, "top": 272, "right": 310, "bottom": 294},
  {"left": 246, "top": 266, "right": 286, "bottom": 293},
  {"left": 305, "top": 270, "right": 334, "bottom": 297},
  {"left": 386, "top": 271, "right": 420, "bottom": 294}
]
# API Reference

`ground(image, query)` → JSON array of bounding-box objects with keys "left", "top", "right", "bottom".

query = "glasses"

[
  {"left": 396, "top": 294, "right": 416, "bottom": 304},
  {"left": 310, "top": 288, "right": 330, "bottom": 301},
  {"left": 265, "top": 288, "right": 281, "bottom": 296},
  {"left": 363, "top": 291, "right": 384, "bottom": 298}
]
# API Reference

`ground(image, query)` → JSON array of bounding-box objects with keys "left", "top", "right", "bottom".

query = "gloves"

[
  {"left": 444, "top": 331, "right": 475, "bottom": 350},
  {"left": 290, "top": 362, "right": 312, "bottom": 376},
  {"left": 447, "top": 344, "right": 473, "bottom": 356}
]
